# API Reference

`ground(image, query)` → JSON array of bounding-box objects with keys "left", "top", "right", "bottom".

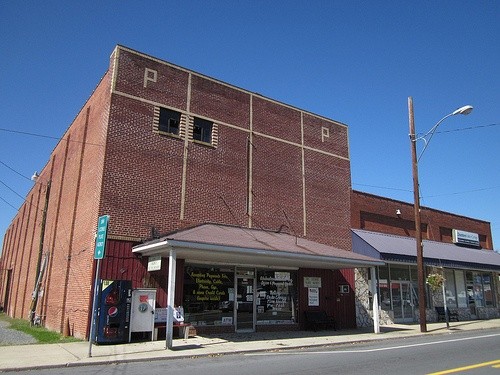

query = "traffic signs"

[{"left": 94, "top": 214, "right": 110, "bottom": 260}]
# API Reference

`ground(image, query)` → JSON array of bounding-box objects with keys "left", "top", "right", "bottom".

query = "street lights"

[{"left": 406, "top": 95, "right": 472, "bottom": 334}]
